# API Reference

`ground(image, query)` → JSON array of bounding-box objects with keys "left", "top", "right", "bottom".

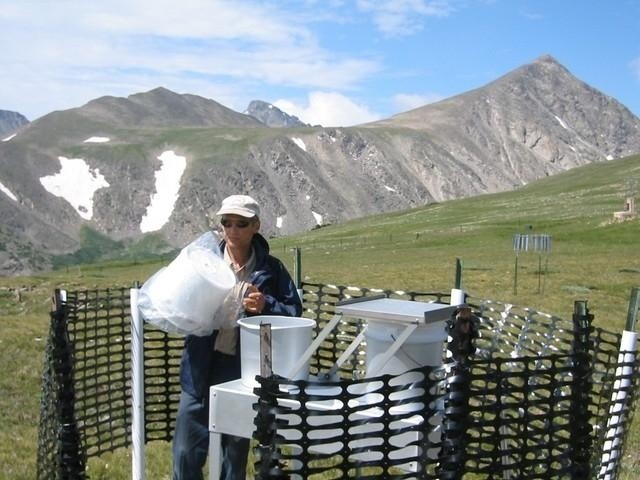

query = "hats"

[{"left": 216, "top": 195, "right": 261, "bottom": 219}]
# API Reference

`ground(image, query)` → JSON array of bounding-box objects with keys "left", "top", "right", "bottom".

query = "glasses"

[{"left": 220, "top": 218, "right": 249, "bottom": 229}]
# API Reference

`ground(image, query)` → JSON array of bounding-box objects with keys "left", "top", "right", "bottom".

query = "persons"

[{"left": 172, "top": 194, "right": 302, "bottom": 480}]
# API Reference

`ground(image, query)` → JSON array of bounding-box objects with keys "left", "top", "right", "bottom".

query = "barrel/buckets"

[
  {"left": 363, "top": 319, "right": 448, "bottom": 412},
  {"left": 149, "top": 246, "right": 236, "bottom": 332},
  {"left": 235, "top": 315, "right": 316, "bottom": 390}
]
